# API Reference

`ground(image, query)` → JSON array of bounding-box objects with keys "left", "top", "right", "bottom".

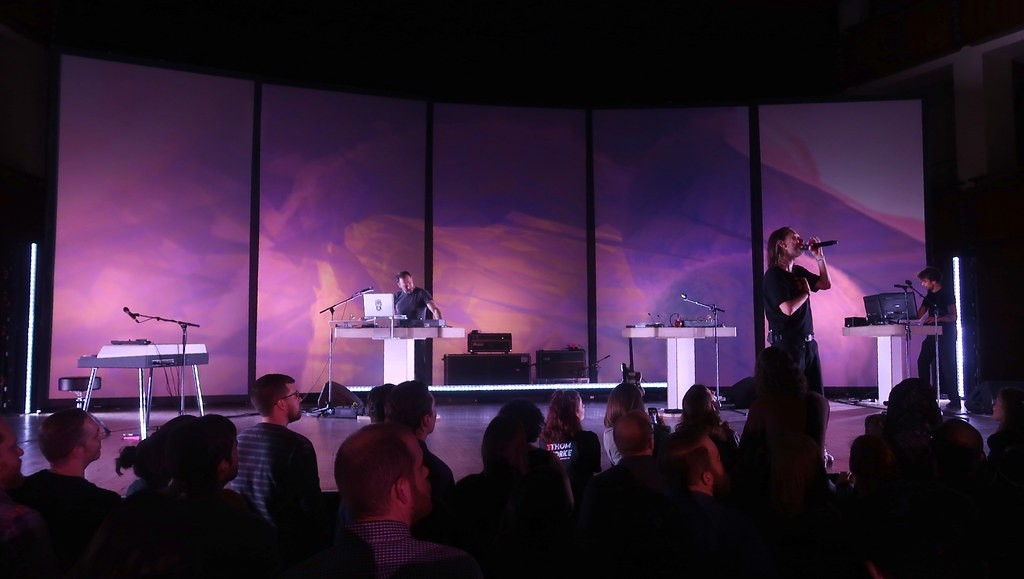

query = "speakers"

[
  {"left": 535, "top": 349, "right": 586, "bottom": 378},
  {"left": 442, "top": 353, "right": 531, "bottom": 385},
  {"left": 318, "top": 380, "right": 365, "bottom": 419},
  {"left": 965, "top": 379, "right": 1024, "bottom": 414}
]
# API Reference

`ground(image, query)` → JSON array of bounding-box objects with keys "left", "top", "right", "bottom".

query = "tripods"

[{"left": 681, "top": 293, "right": 746, "bottom": 418}]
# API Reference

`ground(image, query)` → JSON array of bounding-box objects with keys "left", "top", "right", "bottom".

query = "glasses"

[{"left": 274, "top": 391, "right": 299, "bottom": 405}]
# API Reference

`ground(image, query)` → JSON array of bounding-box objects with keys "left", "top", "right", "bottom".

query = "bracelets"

[{"left": 816, "top": 257, "right": 824, "bottom": 262}]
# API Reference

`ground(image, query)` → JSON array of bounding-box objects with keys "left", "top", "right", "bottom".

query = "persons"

[
  {"left": 917, "top": 267, "right": 961, "bottom": 409},
  {"left": 0, "top": 348, "right": 1024, "bottom": 579},
  {"left": 359, "top": 271, "right": 453, "bottom": 420},
  {"left": 762, "top": 227, "right": 835, "bottom": 468}
]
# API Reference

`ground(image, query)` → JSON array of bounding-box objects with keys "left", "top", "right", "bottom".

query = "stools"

[{"left": 58, "top": 376, "right": 112, "bottom": 435}]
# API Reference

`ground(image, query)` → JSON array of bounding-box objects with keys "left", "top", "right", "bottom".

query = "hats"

[{"left": 917, "top": 268, "right": 942, "bottom": 282}]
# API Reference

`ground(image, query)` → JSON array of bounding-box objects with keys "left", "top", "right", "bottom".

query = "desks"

[
  {"left": 336, "top": 327, "right": 466, "bottom": 423},
  {"left": 842, "top": 325, "right": 944, "bottom": 409},
  {"left": 623, "top": 327, "right": 737, "bottom": 413},
  {"left": 77, "top": 352, "right": 209, "bottom": 441}
]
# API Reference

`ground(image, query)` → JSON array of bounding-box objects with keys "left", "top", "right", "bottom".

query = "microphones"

[
  {"left": 803, "top": 239, "right": 839, "bottom": 250},
  {"left": 894, "top": 284, "right": 909, "bottom": 289},
  {"left": 358, "top": 286, "right": 374, "bottom": 294},
  {"left": 123, "top": 306, "right": 139, "bottom": 323}
]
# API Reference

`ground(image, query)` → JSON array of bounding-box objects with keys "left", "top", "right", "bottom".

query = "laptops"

[
  {"left": 863, "top": 292, "right": 919, "bottom": 325},
  {"left": 364, "top": 293, "right": 406, "bottom": 318}
]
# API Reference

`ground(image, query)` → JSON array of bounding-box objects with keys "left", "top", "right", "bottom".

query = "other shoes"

[{"left": 940, "top": 402, "right": 960, "bottom": 414}]
